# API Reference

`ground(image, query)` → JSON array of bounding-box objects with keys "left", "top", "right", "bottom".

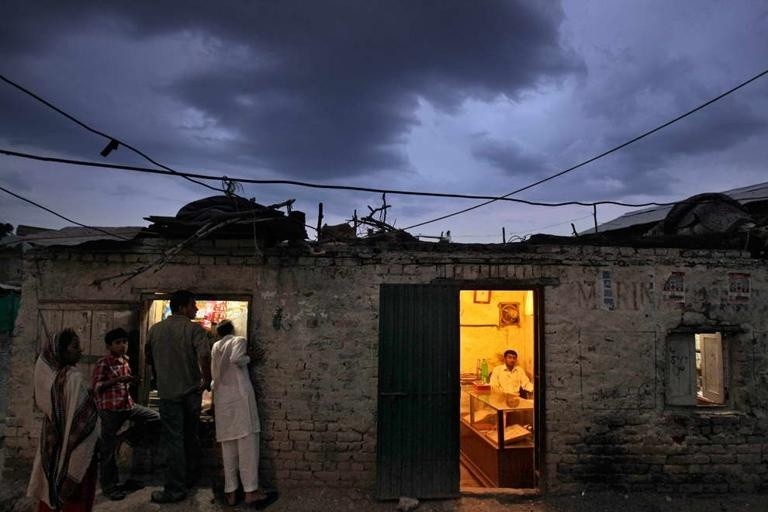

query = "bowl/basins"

[{"left": 472, "top": 382, "right": 490, "bottom": 390}]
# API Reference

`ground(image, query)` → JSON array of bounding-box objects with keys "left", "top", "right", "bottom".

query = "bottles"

[{"left": 476, "top": 359, "right": 488, "bottom": 380}]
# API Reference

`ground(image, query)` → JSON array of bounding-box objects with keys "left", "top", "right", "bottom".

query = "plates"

[{"left": 460, "top": 378, "right": 476, "bottom": 383}]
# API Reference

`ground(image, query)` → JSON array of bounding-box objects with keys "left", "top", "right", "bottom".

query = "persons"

[
  {"left": 92, "top": 328, "right": 160, "bottom": 500},
  {"left": 26, "top": 330, "right": 103, "bottom": 512},
  {"left": 210, "top": 320, "right": 268, "bottom": 506},
  {"left": 145, "top": 290, "right": 211, "bottom": 502},
  {"left": 490, "top": 351, "right": 534, "bottom": 397}
]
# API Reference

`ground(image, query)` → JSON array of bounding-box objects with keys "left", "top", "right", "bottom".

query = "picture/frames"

[
  {"left": 498, "top": 298, "right": 522, "bottom": 331},
  {"left": 473, "top": 287, "right": 492, "bottom": 304}
]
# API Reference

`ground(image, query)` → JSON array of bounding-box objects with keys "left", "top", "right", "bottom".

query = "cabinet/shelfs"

[{"left": 459, "top": 378, "right": 536, "bottom": 489}]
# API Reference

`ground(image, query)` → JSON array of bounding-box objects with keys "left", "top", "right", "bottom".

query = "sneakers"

[
  {"left": 245, "top": 489, "right": 269, "bottom": 504},
  {"left": 151, "top": 487, "right": 197, "bottom": 503},
  {"left": 226, "top": 491, "right": 237, "bottom": 505},
  {"left": 102, "top": 483, "right": 125, "bottom": 500},
  {"left": 117, "top": 478, "right": 145, "bottom": 490}
]
